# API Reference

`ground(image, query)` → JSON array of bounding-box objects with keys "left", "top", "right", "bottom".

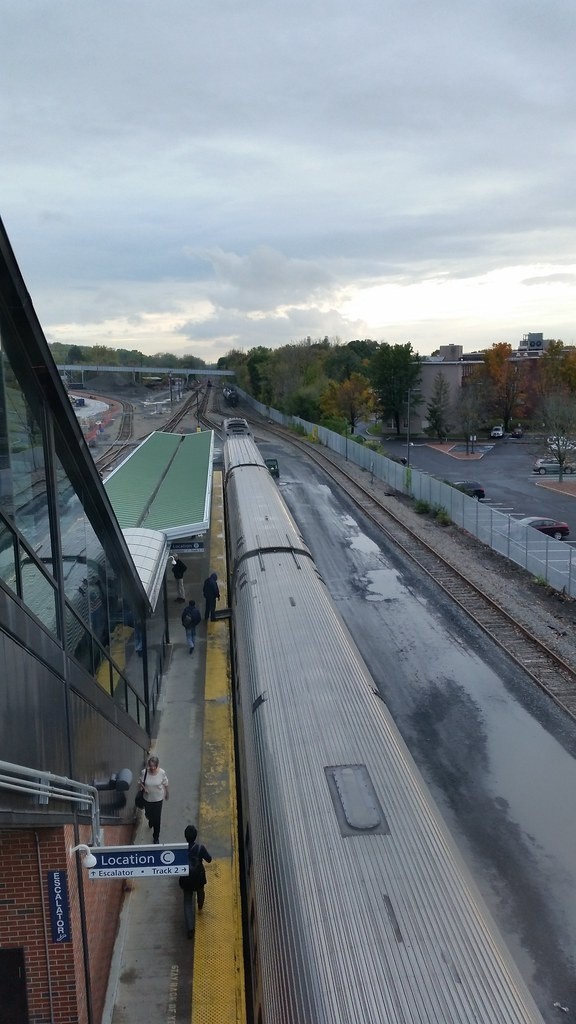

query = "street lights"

[{"left": 406, "top": 388, "right": 421, "bottom": 468}]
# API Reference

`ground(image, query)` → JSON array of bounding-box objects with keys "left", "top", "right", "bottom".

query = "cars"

[
  {"left": 513, "top": 427, "right": 522, "bottom": 437},
  {"left": 390, "top": 455, "right": 407, "bottom": 466},
  {"left": 548, "top": 442, "right": 576, "bottom": 453},
  {"left": 503, "top": 517, "right": 569, "bottom": 540},
  {"left": 546, "top": 437, "right": 569, "bottom": 445}
]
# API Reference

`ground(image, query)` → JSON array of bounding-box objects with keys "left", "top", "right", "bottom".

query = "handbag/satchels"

[
  {"left": 184, "top": 613, "right": 193, "bottom": 628},
  {"left": 134, "top": 790, "right": 145, "bottom": 810}
]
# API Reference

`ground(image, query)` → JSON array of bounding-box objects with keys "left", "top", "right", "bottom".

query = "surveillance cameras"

[
  {"left": 172, "top": 561, "right": 177, "bottom": 565},
  {"left": 83, "top": 854, "right": 97, "bottom": 869}
]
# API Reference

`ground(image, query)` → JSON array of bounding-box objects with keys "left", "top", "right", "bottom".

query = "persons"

[
  {"left": 182, "top": 599, "right": 200, "bottom": 656},
  {"left": 203, "top": 574, "right": 221, "bottom": 624},
  {"left": 138, "top": 756, "right": 169, "bottom": 844},
  {"left": 171, "top": 553, "right": 188, "bottom": 604},
  {"left": 179, "top": 824, "right": 213, "bottom": 938}
]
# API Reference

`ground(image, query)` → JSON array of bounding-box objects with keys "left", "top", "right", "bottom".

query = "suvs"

[
  {"left": 453, "top": 481, "right": 485, "bottom": 501},
  {"left": 533, "top": 460, "right": 576, "bottom": 474},
  {"left": 491, "top": 427, "right": 504, "bottom": 437},
  {"left": 265, "top": 458, "right": 278, "bottom": 477}
]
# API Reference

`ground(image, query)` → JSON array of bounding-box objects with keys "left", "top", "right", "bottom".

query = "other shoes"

[
  {"left": 204, "top": 616, "right": 209, "bottom": 620},
  {"left": 154, "top": 839, "right": 159, "bottom": 844},
  {"left": 211, "top": 618, "right": 217, "bottom": 622},
  {"left": 178, "top": 599, "right": 185, "bottom": 603},
  {"left": 190, "top": 647, "right": 194, "bottom": 654},
  {"left": 175, "top": 598, "right": 182, "bottom": 602},
  {"left": 149, "top": 822, "right": 152, "bottom": 827},
  {"left": 198, "top": 904, "right": 203, "bottom": 909},
  {"left": 187, "top": 929, "right": 195, "bottom": 940}
]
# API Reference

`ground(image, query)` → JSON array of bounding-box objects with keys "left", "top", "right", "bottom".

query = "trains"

[
  {"left": 224, "top": 387, "right": 239, "bottom": 404},
  {"left": 221, "top": 418, "right": 576, "bottom": 1024}
]
